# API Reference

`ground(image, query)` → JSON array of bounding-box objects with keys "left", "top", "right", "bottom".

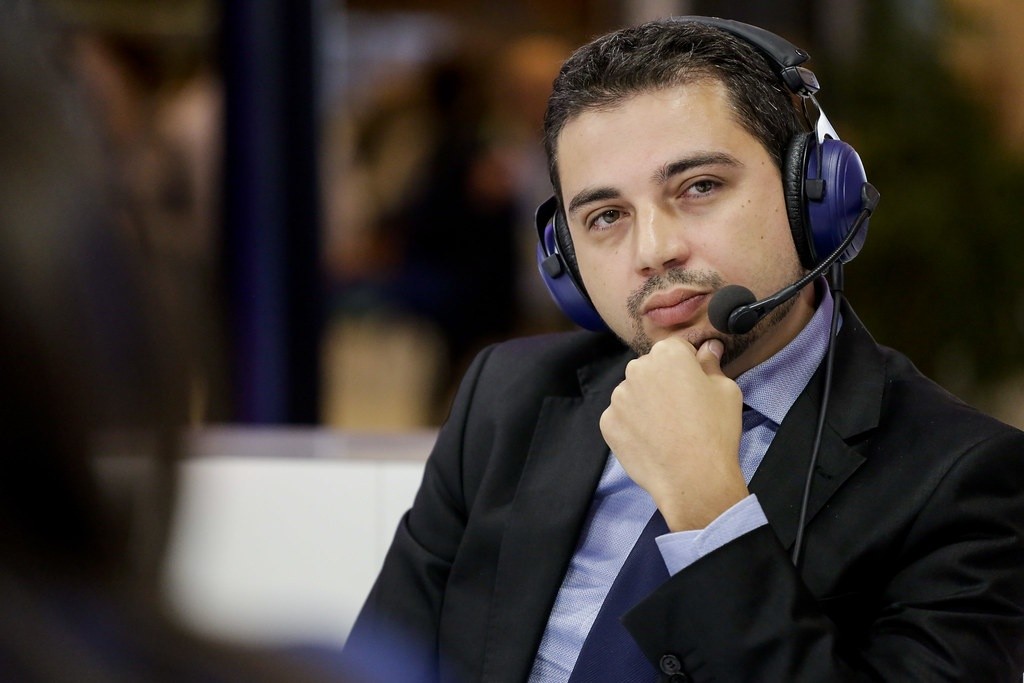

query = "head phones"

[{"left": 534, "top": 16, "right": 881, "bottom": 337}]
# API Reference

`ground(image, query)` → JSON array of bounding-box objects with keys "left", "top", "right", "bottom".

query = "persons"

[
  {"left": 0, "top": 0, "right": 342, "bottom": 683},
  {"left": 342, "top": 15, "right": 1024, "bottom": 682}
]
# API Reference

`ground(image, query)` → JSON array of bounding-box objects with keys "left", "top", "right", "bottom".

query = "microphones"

[{"left": 707, "top": 182, "right": 880, "bottom": 338}]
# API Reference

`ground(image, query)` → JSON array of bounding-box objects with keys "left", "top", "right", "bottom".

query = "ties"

[{"left": 567, "top": 403, "right": 766, "bottom": 683}]
